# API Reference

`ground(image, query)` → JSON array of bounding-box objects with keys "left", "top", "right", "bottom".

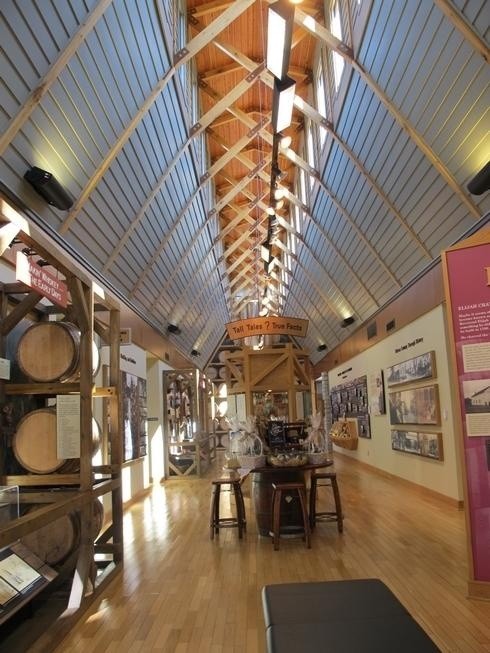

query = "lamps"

[
  {"left": 193, "top": 349, "right": 198, "bottom": 356},
  {"left": 338, "top": 317, "right": 356, "bottom": 330},
  {"left": 27, "top": 166, "right": 73, "bottom": 210},
  {"left": 317, "top": 344, "right": 327, "bottom": 350},
  {"left": 168, "top": 323, "right": 181, "bottom": 336},
  {"left": 467, "top": 159, "right": 490, "bottom": 195}
]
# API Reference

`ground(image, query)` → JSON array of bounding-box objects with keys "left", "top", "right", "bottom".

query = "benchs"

[{"left": 262, "top": 578, "right": 443, "bottom": 653}]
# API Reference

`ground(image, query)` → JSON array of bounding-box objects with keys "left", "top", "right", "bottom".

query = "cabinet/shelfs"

[
  {"left": 0, "top": 203, "right": 123, "bottom": 628},
  {"left": 164, "top": 369, "right": 214, "bottom": 481}
]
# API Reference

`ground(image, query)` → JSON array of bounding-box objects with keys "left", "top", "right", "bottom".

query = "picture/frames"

[
  {"left": 385, "top": 352, "right": 436, "bottom": 387},
  {"left": 102, "top": 365, "right": 147, "bottom": 467},
  {"left": 386, "top": 384, "right": 442, "bottom": 425},
  {"left": 391, "top": 430, "right": 444, "bottom": 459}
]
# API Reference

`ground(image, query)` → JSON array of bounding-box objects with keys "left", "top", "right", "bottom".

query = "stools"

[
  {"left": 208, "top": 479, "right": 247, "bottom": 541},
  {"left": 269, "top": 483, "right": 312, "bottom": 551},
  {"left": 310, "top": 473, "right": 342, "bottom": 535}
]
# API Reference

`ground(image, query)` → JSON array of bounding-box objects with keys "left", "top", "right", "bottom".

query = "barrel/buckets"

[
  {"left": 19, "top": 488, "right": 104, "bottom": 569},
  {"left": 16, "top": 322, "right": 100, "bottom": 383},
  {"left": 12, "top": 405, "right": 102, "bottom": 473}
]
